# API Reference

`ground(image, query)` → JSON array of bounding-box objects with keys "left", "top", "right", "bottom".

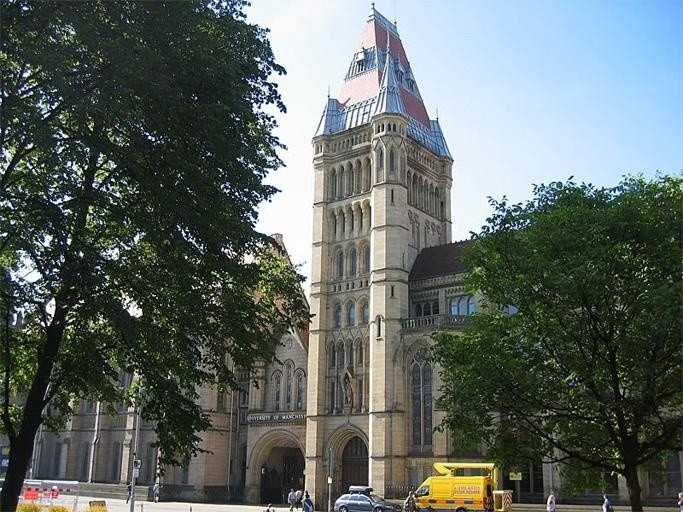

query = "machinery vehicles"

[{"left": 403, "top": 462, "right": 513, "bottom": 512}]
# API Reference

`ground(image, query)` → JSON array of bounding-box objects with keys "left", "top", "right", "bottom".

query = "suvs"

[{"left": 333, "top": 485, "right": 402, "bottom": 512}]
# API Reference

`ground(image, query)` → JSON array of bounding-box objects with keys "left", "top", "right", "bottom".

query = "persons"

[
  {"left": 125, "top": 480, "right": 135, "bottom": 503},
  {"left": 405, "top": 491, "right": 417, "bottom": 512},
  {"left": 300, "top": 490, "right": 309, "bottom": 510},
  {"left": 151, "top": 482, "right": 163, "bottom": 502},
  {"left": 677, "top": 492, "right": 683, "bottom": 512},
  {"left": 294, "top": 486, "right": 302, "bottom": 508},
  {"left": 547, "top": 490, "right": 556, "bottom": 512},
  {"left": 600, "top": 492, "right": 610, "bottom": 512},
  {"left": 287, "top": 488, "right": 297, "bottom": 512}
]
